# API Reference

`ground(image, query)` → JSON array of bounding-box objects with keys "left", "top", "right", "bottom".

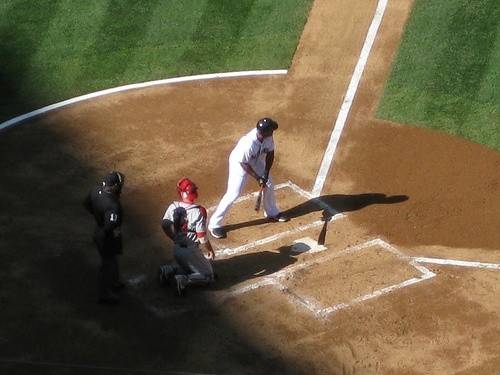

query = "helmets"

[
  {"left": 177, "top": 179, "right": 198, "bottom": 200},
  {"left": 257, "top": 118, "right": 278, "bottom": 132}
]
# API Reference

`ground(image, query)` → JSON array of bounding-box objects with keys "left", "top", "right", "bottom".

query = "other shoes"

[
  {"left": 111, "top": 282, "right": 126, "bottom": 291},
  {"left": 99, "top": 297, "right": 120, "bottom": 304}
]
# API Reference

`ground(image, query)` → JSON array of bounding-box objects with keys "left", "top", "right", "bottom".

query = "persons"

[
  {"left": 158, "top": 178, "right": 215, "bottom": 296},
  {"left": 208, "top": 117, "right": 288, "bottom": 239},
  {"left": 82, "top": 170, "right": 126, "bottom": 305}
]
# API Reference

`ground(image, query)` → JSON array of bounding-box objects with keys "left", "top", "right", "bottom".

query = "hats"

[{"left": 102, "top": 172, "right": 122, "bottom": 186}]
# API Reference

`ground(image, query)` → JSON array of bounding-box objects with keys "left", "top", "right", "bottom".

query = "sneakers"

[
  {"left": 208, "top": 228, "right": 225, "bottom": 239},
  {"left": 159, "top": 266, "right": 169, "bottom": 285},
  {"left": 174, "top": 274, "right": 186, "bottom": 295},
  {"left": 264, "top": 211, "right": 287, "bottom": 222}
]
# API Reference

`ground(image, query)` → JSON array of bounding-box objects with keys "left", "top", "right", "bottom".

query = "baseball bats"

[{"left": 255, "top": 183, "right": 264, "bottom": 211}]
